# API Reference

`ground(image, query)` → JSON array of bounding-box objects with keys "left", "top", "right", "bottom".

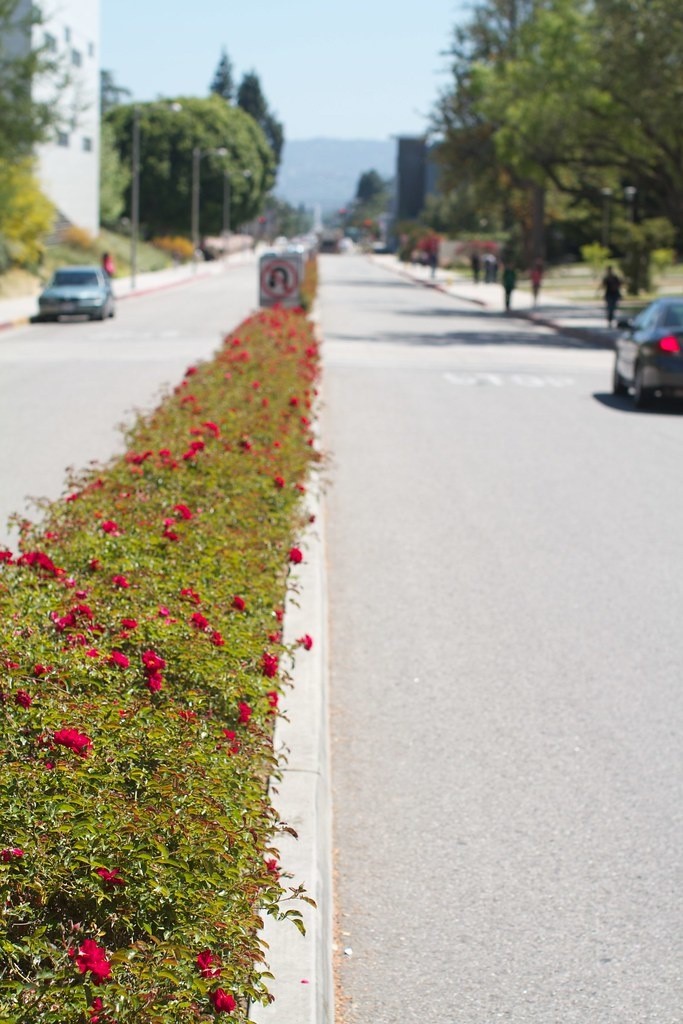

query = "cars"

[
  {"left": 36, "top": 263, "right": 117, "bottom": 321},
  {"left": 609, "top": 294, "right": 683, "bottom": 412}
]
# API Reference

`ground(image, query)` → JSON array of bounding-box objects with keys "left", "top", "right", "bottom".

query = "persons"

[
  {"left": 470, "top": 253, "right": 480, "bottom": 282},
  {"left": 102, "top": 251, "right": 115, "bottom": 276},
  {"left": 484, "top": 261, "right": 498, "bottom": 283},
  {"left": 597, "top": 265, "right": 622, "bottom": 325},
  {"left": 501, "top": 262, "right": 516, "bottom": 310},
  {"left": 429, "top": 252, "right": 438, "bottom": 280},
  {"left": 529, "top": 259, "right": 543, "bottom": 306}
]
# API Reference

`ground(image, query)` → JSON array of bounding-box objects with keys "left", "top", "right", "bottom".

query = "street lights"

[
  {"left": 130, "top": 102, "right": 182, "bottom": 291},
  {"left": 191, "top": 144, "right": 229, "bottom": 257},
  {"left": 222, "top": 165, "right": 255, "bottom": 249}
]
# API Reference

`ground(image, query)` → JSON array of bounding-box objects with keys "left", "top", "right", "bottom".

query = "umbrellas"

[{"left": 481, "top": 253, "right": 496, "bottom": 263}]
